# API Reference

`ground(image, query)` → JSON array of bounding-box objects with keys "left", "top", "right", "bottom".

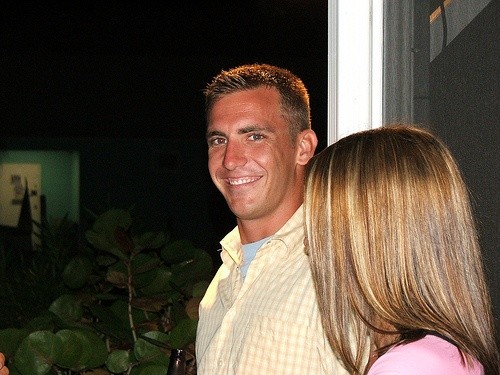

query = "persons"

[
  {"left": 299, "top": 120, "right": 500, "bottom": 375},
  {"left": 193, "top": 60, "right": 359, "bottom": 375}
]
